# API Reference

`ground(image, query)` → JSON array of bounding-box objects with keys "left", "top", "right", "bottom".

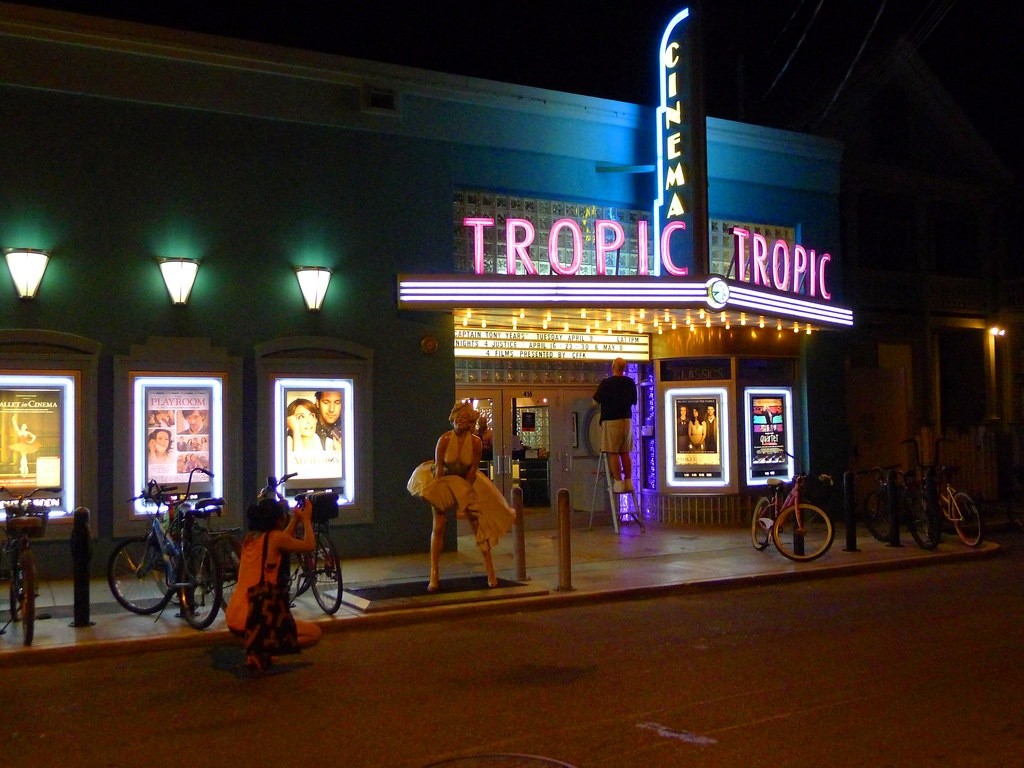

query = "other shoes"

[
  {"left": 612, "top": 485, "right": 623, "bottom": 493},
  {"left": 624, "top": 485, "right": 633, "bottom": 492}
]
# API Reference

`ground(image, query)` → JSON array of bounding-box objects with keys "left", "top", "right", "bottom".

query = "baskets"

[
  {"left": 5, "top": 505, "right": 51, "bottom": 538},
  {"left": 801, "top": 473, "right": 830, "bottom": 503}
]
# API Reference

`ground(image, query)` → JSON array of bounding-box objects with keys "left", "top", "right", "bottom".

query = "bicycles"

[
  {"left": 148, "top": 468, "right": 243, "bottom": 613},
  {"left": 857, "top": 462, "right": 937, "bottom": 552},
  {"left": 751, "top": 461, "right": 836, "bottom": 562},
  {"left": 255, "top": 472, "right": 343, "bottom": 615},
  {"left": 0, "top": 485, "right": 62, "bottom": 646},
  {"left": 108, "top": 485, "right": 224, "bottom": 630},
  {"left": 921, "top": 463, "right": 985, "bottom": 549}
]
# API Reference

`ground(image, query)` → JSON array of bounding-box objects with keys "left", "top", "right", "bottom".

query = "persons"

[
  {"left": 226, "top": 499, "right": 322, "bottom": 678},
  {"left": 407, "top": 402, "right": 516, "bottom": 592},
  {"left": 9, "top": 417, "right": 38, "bottom": 474},
  {"left": 592, "top": 358, "right": 637, "bottom": 492},
  {"left": 474, "top": 417, "right": 493, "bottom": 460},
  {"left": 286, "top": 390, "right": 341, "bottom": 452},
  {"left": 677, "top": 405, "right": 717, "bottom": 451},
  {"left": 147, "top": 410, "right": 208, "bottom": 474}
]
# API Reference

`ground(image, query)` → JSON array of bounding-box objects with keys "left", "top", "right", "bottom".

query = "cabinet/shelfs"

[{"left": 517, "top": 405, "right": 549, "bottom": 451}]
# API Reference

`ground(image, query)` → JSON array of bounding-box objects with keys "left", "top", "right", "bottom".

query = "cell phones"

[{"left": 298, "top": 498, "right": 305, "bottom": 511}]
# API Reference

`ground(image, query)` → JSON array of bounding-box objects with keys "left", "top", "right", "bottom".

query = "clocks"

[{"left": 709, "top": 278, "right": 730, "bottom": 304}]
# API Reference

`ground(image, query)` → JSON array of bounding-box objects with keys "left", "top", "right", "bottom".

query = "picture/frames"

[{"left": 573, "top": 412, "right": 578, "bottom": 448}]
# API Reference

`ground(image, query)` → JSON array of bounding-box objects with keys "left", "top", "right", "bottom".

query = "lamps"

[
  {"left": 2, "top": 247, "right": 54, "bottom": 300},
  {"left": 296, "top": 265, "right": 334, "bottom": 313},
  {"left": 156, "top": 256, "right": 202, "bottom": 305}
]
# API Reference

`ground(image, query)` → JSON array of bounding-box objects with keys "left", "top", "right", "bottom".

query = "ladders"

[{"left": 586, "top": 450, "right": 644, "bottom": 536}]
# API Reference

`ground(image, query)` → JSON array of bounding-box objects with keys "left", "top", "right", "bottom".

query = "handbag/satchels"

[{"left": 244, "top": 530, "right": 301, "bottom": 657}]
